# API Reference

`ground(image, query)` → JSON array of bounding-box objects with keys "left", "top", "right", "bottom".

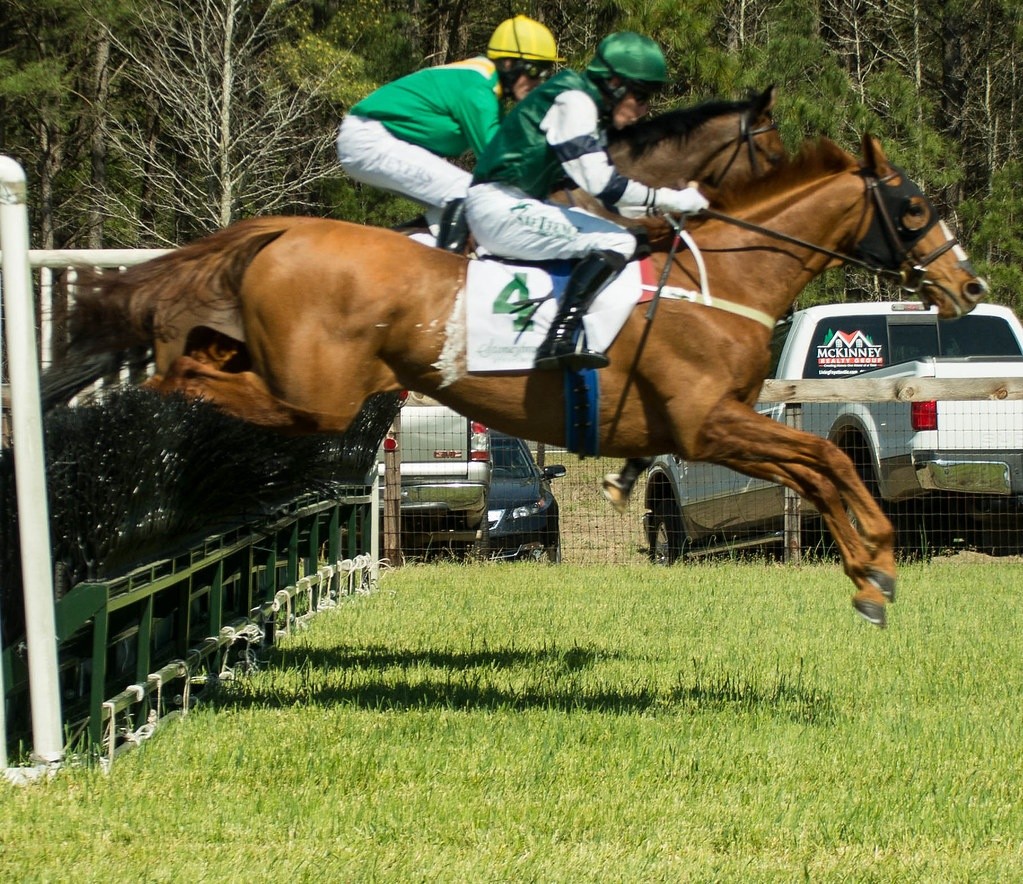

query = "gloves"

[{"left": 655, "top": 186, "right": 709, "bottom": 217}]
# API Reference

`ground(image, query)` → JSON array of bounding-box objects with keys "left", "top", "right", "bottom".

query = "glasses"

[
  {"left": 626, "top": 82, "right": 654, "bottom": 103},
  {"left": 515, "top": 60, "right": 553, "bottom": 80}
]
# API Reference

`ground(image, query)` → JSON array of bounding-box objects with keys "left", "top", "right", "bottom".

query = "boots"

[
  {"left": 532, "top": 255, "right": 610, "bottom": 373},
  {"left": 435, "top": 195, "right": 469, "bottom": 253}
]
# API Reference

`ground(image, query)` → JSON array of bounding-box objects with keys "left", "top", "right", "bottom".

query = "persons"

[
  {"left": 337, "top": 9, "right": 570, "bottom": 251},
  {"left": 463, "top": 26, "right": 713, "bottom": 369}
]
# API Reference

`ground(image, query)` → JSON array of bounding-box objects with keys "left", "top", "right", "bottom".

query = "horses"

[
  {"left": 58, "top": 138, "right": 994, "bottom": 627},
  {"left": 394, "top": 84, "right": 791, "bottom": 508}
]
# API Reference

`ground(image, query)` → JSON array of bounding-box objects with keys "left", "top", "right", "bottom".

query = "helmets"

[
  {"left": 485, "top": 14, "right": 568, "bottom": 64},
  {"left": 586, "top": 30, "right": 672, "bottom": 85}
]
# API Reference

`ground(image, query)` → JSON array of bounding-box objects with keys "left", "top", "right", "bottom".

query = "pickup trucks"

[
  {"left": 377, "top": 389, "right": 491, "bottom": 556},
  {"left": 644, "top": 296, "right": 1023, "bottom": 568}
]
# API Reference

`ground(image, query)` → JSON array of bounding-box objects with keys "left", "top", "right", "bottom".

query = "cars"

[{"left": 488, "top": 435, "right": 567, "bottom": 559}]
